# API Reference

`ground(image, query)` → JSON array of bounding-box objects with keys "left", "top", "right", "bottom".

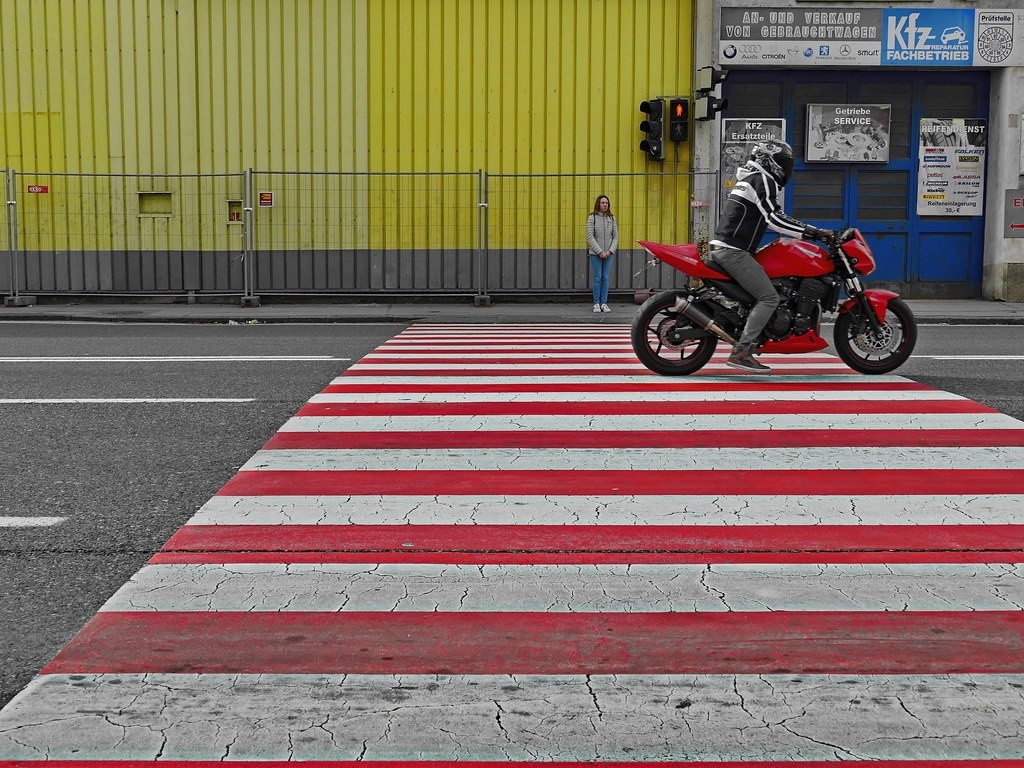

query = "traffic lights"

[
  {"left": 638, "top": 98, "right": 665, "bottom": 159},
  {"left": 670, "top": 99, "right": 690, "bottom": 141}
]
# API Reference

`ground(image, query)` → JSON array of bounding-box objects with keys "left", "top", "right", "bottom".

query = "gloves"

[{"left": 802, "top": 224, "right": 837, "bottom": 245}]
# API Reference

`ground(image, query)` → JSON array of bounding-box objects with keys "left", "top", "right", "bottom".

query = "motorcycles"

[{"left": 629, "top": 229, "right": 919, "bottom": 378}]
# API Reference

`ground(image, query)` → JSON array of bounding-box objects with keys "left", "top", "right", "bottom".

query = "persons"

[
  {"left": 710, "top": 138, "right": 836, "bottom": 374},
  {"left": 586, "top": 195, "right": 619, "bottom": 312}
]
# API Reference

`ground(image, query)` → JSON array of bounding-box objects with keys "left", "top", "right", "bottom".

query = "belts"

[{"left": 710, "top": 244, "right": 731, "bottom": 251}]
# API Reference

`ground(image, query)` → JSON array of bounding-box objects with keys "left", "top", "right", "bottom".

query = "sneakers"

[
  {"left": 593, "top": 303, "right": 611, "bottom": 313},
  {"left": 727, "top": 342, "right": 771, "bottom": 373}
]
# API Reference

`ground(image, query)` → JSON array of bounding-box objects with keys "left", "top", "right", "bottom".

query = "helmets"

[{"left": 748, "top": 139, "right": 795, "bottom": 188}]
